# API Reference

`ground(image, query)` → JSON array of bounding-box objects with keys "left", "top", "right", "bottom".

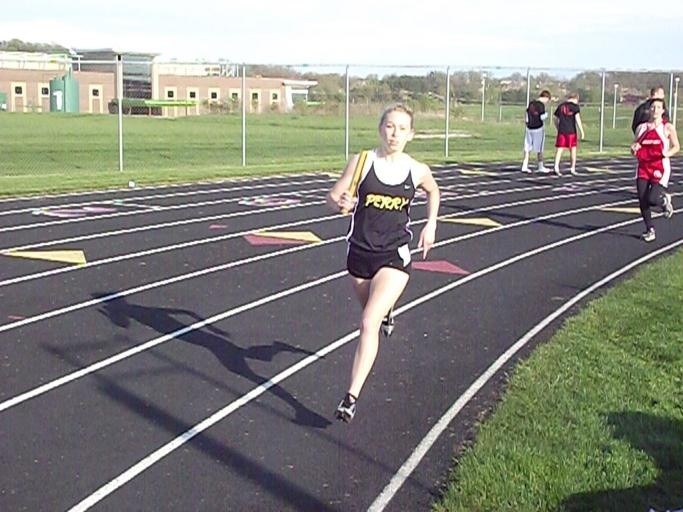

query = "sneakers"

[
  {"left": 380, "top": 308, "right": 395, "bottom": 337},
  {"left": 661, "top": 193, "right": 673, "bottom": 220},
  {"left": 641, "top": 228, "right": 655, "bottom": 242},
  {"left": 334, "top": 392, "right": 357, "bottom": 424},
  {"left": 521, "top": 168, "right": 580, "bottom": 176}
]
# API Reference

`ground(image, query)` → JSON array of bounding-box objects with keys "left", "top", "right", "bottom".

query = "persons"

[
  {"left": 553, "top": 92, "right": 585, "bottom": 177},
  {"left": 630, "top": 84, "right": 680, "bottom": 242},
  {"left": 327, "top": 106, "right": 441, "bottom": 424},
  {"left": 521, "top": 90, "right": 552, "bottom": 174}
]
